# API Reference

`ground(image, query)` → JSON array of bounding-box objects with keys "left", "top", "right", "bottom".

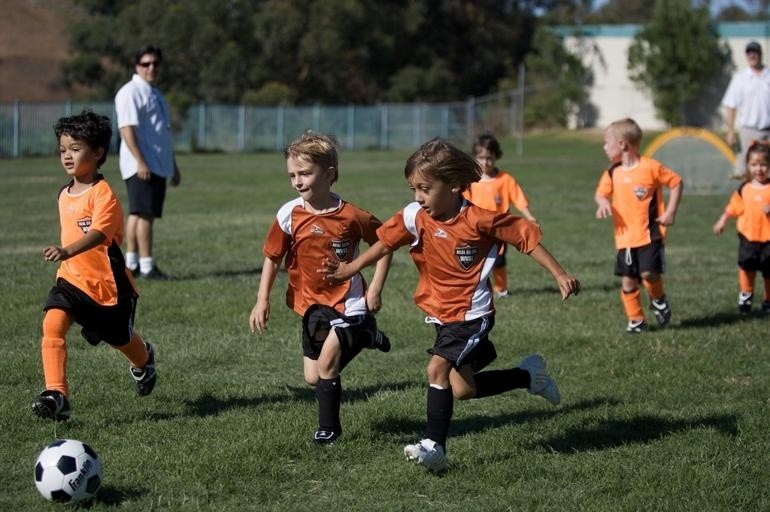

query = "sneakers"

[
  {"left": 30, "top": 390, "right": 70, "bottom": 421},
  {"left": 760, "top": 301, "right": 770, "bottom": 313},
  {"left": 375, "top": 329, "right": 391, "bottom": 352},
  {"left": 130, "top": 340, "right": 157, "bottom": 396},
  {"left": 626, "top": 320, "right": 647, "bottom": 334},
  {"left": 404, "top": 439, "right": 447, "bottom": 474},
  {"left": 497, "top": 291, "right": 510, "bottom": 299},
  {"left": 738, "top": 291, "right": 752, "bottom": 316},
  {"left": 128, "top": 263, "right": 140, "bottom": 278},
  {"left": 518, "top": 353, "right": 561, "bottom": 407},
  {"left": 141, "top": 264, "right": 177, "bottom": 282},
  {"left": 649, "top": 295, "right": 671, "bottom": 327},
  {"left": 315, "top": 430, "right": 336, "bottom": 442}
]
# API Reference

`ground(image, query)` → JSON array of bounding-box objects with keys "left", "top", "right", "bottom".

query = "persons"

[
  {"left": 114, "top": 45, "right": 181, "bottom": 280},
  {"left": 31, "top": 109, "right": 156, "bottom": 421},
  {"left": 722, "top": 42, "right": 770, "bottom": 183},
  {"left": 316, "top": 137, "right": 582, "bottom": 473},
  {"left": 594, "top": 119, "right": 684, "bottom": 332},
  {"left": 461, "top": 133, "right": 540, "bottom": 298},
  {"left": 713, "top": 139, "right": 770, "bottom": 314},
  {"left": 249, "top": 130, "right": 393, "bottom": 446}
]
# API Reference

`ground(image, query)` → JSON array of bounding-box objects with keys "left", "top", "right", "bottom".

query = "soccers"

[{"left": 33, "top": 440, "right": 102, "bottom": 504}]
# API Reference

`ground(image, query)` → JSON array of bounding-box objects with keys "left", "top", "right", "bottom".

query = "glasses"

[{"left": 138, "top": 61, "right": 159, "bottom": 67}]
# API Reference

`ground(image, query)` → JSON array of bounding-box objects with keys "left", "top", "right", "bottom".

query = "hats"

[{"left": 746, "top": 42, "right": 760, "bottom": 52}]
